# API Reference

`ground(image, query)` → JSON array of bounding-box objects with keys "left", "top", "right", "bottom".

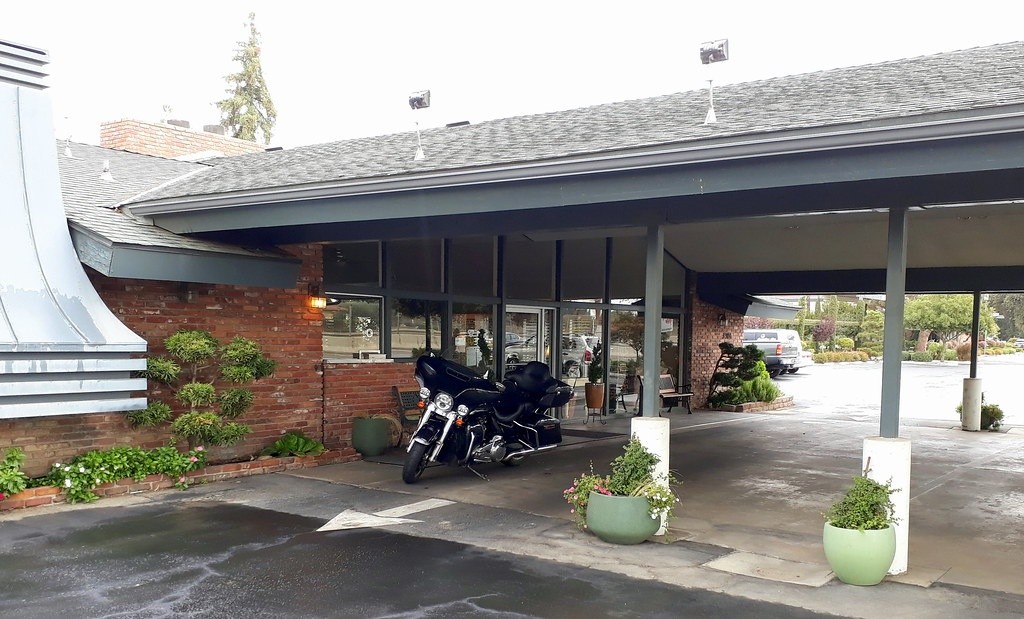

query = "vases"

[{"left": 587, "top": 491, "right": 661, "bottom": 546}]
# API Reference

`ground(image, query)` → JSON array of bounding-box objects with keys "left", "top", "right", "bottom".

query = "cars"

[
  {"left": 505, "top": 333, "right": 604, "bottom": 377},
  {"left": 1014, "top": 339, "right": 1024, "bottom": 347},
  {"left": 788, "top": 351, "right": 814, "bottom": 373}
]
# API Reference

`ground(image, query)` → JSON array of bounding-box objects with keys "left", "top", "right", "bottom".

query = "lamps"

[
  {"left": 697, "top": 39, "right": 728, "bottom": 129},
  {"left": 182, "top": 282, "right": 200, "bottom": 305},
  {"left": 305, "top": 281, "right": 327, "bottom": 310},
  {"left": 409, "top": 89, "right": 430, "bottom": 162}
]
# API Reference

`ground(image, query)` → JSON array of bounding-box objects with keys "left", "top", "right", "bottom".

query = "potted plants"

[
  {"left": 957, "top": 403, "right": 1004, "bottom": 430},
  {"left": 822, "top": 476, "right": 900, "bottom": 581},
  {"left": 351, "top": 412, "right": 402, "bottom": 456},
  {"left": 583, "top": 339, "right": 607, "bottom": 427},
  {"left": 721, "top": 386, "right": 744, "bottom": 413}
]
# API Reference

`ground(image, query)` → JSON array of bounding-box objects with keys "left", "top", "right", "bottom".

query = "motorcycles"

[{"left": 402, "top": 350, "right": 575, "bottom": 483}]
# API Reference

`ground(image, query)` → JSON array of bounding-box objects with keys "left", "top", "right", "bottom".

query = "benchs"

[
  {"left": 393, "top": 383, "right": 427, "bottom": 447},
  {"left": 635, "top": 374, "right": 695, "bottom": 418}
]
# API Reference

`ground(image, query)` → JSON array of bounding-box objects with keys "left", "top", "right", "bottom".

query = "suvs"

[{"left": 743, "top": 328, "right": 802, "bottom": 376}]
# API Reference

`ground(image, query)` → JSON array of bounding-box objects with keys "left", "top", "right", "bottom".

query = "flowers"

[{"left": 558, "top": 466, "right": 681, "bottom": 530}]
